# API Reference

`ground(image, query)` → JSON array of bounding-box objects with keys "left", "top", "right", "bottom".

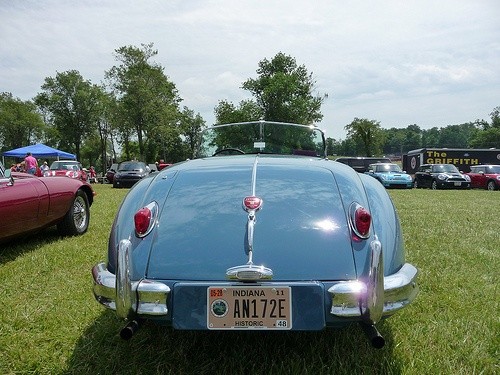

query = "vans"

[{"left": 335, "top": 157, "right": 392, "bottom": 173}]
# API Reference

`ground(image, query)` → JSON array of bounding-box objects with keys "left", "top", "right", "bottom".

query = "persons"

[
  {"left": 449, "top": 160, "right": 467, "bottom": 174},
  {"left": 155, "top": 159, "right": 172, "bottom": 171},
  {"left": 11, "top": 152, "right": 50, "bottom": 176},
  {"left": 82, "top": 165, "right": 115, "bottom": 184}
]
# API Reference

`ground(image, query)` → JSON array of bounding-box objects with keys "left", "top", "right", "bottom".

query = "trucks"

[{"left": 403, "top": 147, "right": 500, "bottom": 175}]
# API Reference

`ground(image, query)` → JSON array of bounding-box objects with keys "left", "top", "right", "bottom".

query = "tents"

[{"left": 3, "top": 143, "right": 76, "bottom": 166}]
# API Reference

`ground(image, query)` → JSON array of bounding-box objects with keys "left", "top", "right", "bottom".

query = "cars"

[
  {"left": 463, "top": 164, "right": 500, "bottom": 191},
  {"left": 92, "top": 114, "right": 419, "bottom": 351},
  {"left": 0, "top": 168, "right": 95, "bottom": 243},
  {"left": 106, "top": 164, "right": 118, "bottom": 184},
  {"left": 364, "top": 163, "right": 412, "bottom": 189},
  {"left": 158, "top": 164, "right": 171, "bottom": 170},
  {"left": 43, "top": 160, "right": 87, "bottom": 182},
  {"left": 0, "top": 163, "right": 37, "bottom": 179},
  {"left": 110, "top": 160, "right": 159, "bottom": 188},
  {"left": 412, "top": 163, "right": 471, "bottom": 190}
]
